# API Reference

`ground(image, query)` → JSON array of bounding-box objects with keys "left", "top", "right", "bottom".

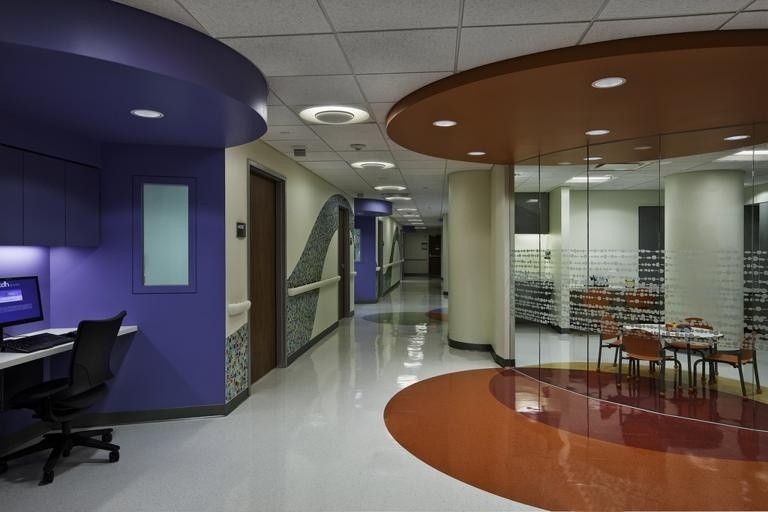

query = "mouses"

[{"left": 67, "top": 331, "right": 77, "bottom": 336}]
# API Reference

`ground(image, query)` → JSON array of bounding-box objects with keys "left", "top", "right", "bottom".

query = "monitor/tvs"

[{"left": 0, "top": 276, "right": 43, "bottom": 327}]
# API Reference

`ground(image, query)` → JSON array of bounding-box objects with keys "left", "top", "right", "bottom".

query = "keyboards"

[{"left": 0, "top": 333, "right": 72, "bottom": 353}]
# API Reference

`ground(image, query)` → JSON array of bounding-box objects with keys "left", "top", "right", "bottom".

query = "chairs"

[
  {"left": 581, "top": 287, "right": 762, "bottom": 401},
  {"left": 0, "top": 309, "right": 127, "bottom": 486}
]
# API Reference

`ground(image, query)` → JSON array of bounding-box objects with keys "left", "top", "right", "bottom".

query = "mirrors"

[{"left": 132, "top": 174, "right": 197, "bottom": 294}]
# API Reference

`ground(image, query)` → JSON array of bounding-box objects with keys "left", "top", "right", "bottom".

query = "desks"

[{"left": 0, "top": 326, "right": 139, "bottom": 370}]
